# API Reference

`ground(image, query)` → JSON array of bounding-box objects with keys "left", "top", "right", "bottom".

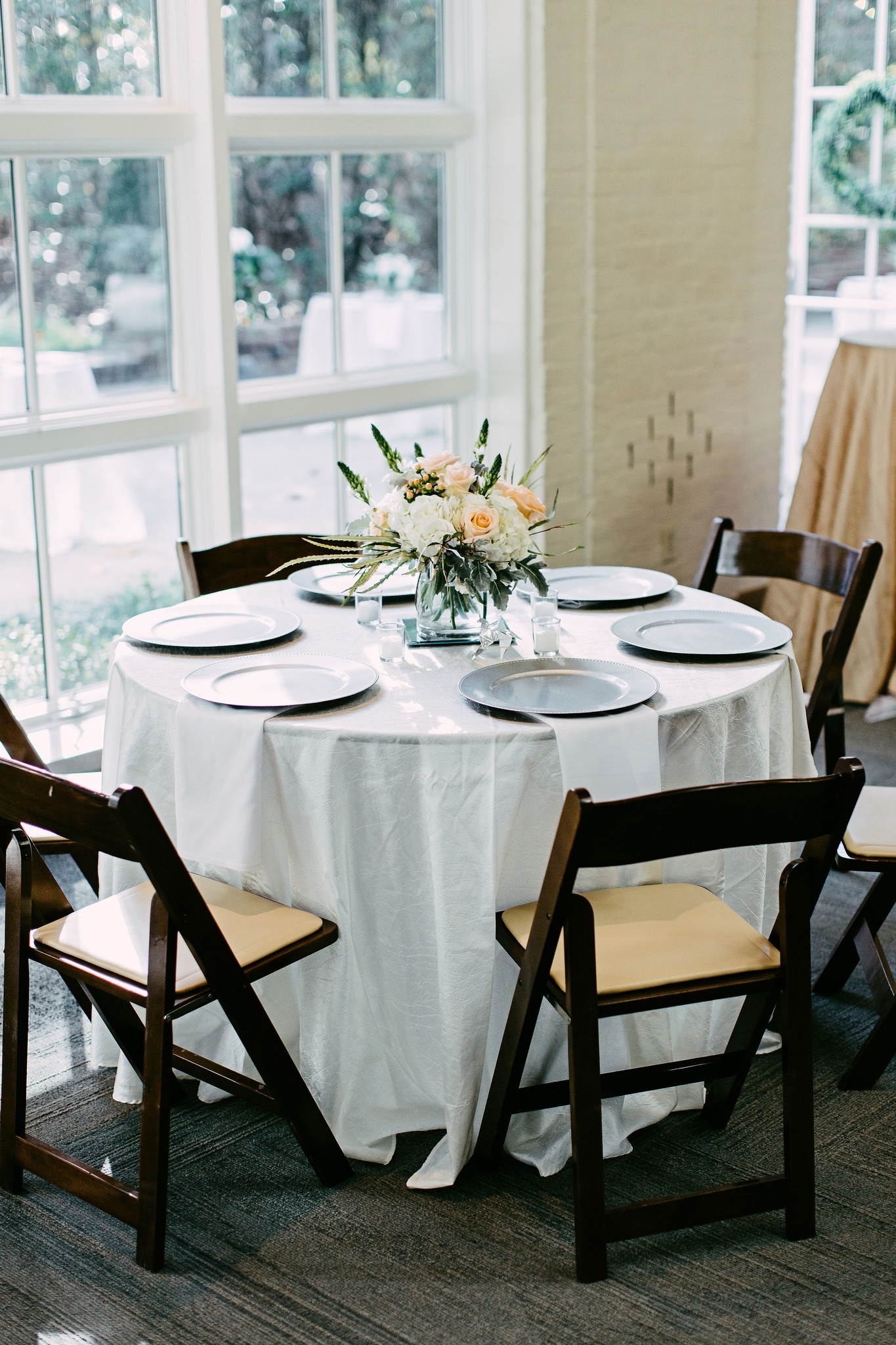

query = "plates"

[
  {"left": 513, "top": 565, "right": 678, "bottom": 601},
  {"left": 287, "top": 560, "right": 426, "bottom": 598},
  {"left": 610, "top": 608, "right": 793, "bottom": 655},
  {"left": 121, "top": 602, "right": 302, "bottom": 649},
  {"left": 178, "top": 650, "right": 377, "bottom": 707},
  {"left": 457, "top": 655, "right": 661, "bottom": 716}
]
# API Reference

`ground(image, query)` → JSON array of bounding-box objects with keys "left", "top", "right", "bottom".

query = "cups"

[
  {"left": 376, "top": 621, "right": 407, "bottom": 663},
  {"left": 531, "top": 587, "right": 558, "bottom": 624},
  {"left": 355, "top": 587, "right": 383, "bottom": 625},
  {"left": 531, "top": 616, "right": 561, "bottom": 657}
]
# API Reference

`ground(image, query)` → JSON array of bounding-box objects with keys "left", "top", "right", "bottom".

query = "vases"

[{"left": 413, "top": 562, "right": 493, "bottom": 645}]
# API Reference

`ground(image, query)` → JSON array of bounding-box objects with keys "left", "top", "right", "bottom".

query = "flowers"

[{"left": 267, "top": 414, "right": 588, "bottom": 626}]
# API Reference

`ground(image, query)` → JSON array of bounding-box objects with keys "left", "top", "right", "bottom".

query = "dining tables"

[{"left": 89, "top": 559, "right": 831, "bottom": 1156}]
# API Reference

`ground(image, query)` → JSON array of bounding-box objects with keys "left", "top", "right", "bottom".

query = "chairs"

[
  {"left": 691, "top": 513, "right": 884, "bottom": 777},
  {"left": 813, "top": 779, "right": 896, "bottom": 1095},
  {"left": 1, "top": 693, "right": 112, "bottom": 919},
  {"left": 0, "top": 756, "right": 356, "bottom": 1275},
  {"left": 175, "top": 532, "right": 363, "bottom": 598},
  {"left": 465, "top": 756, "right": 868, "bottom": 1284}
]
